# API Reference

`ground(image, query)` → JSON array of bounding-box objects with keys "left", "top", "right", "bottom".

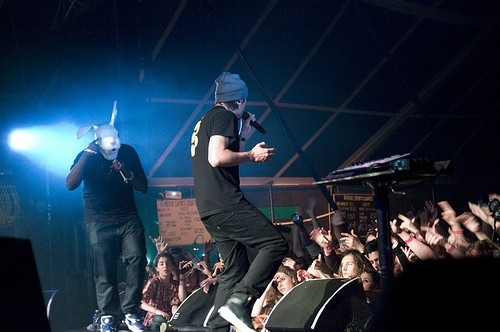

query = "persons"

[
  {"left": 138, "top": 192, "right": 500, "bottom": 332},
  {"left": 188, "top": 70, "right": 289, "bottom": 332},
  {"left": 65, "top": 123, "right": 148, "bottom": 332}
]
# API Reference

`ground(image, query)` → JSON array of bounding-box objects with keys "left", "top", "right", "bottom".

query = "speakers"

[
  {"left": 266, "top": 276, "right": 367, "bottom": 332},
  {"left": 165, "top": 279, "right": 219, "bottom": 332},
  {"left": 372, "top": 254, "right": 500, "bottom": 332}
]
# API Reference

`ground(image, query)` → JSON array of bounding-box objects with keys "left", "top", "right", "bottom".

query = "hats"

[{"left": 214, "top": 72, "right": 249, "bottom": 103}]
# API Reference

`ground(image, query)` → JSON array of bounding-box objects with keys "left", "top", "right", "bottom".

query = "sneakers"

[
  {"left": 218, "top": 292, "right": 258, "bottom": 332},
  {"left": 124, "top": 314, "right": 147, "bottom": 332},
  {"left": 99, "top": 316, "right": 117, "bottom": 332}
]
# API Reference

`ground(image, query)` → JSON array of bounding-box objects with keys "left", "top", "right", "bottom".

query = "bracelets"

[
  {"left": 448, "top": 246, "right": 456, "bottom": 253},
  {"left": 452, "top": 228, "right": 464, "bottom": 234},
  {"left": 324, "top": 250, "right": 332, "bottom": 253},
  {"left": 204, "top": 252, "right": 210, "bottom": 255},
  {"left": 402, "top": 235, "right": 414, "bottom": 243},
  {"left": 178, "top": 277, "right": 186, "bottom": 281}
]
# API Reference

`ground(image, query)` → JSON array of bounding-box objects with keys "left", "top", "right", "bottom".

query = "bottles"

[{"left": 93, "top": 310, "right": 100, "bottom": 331}]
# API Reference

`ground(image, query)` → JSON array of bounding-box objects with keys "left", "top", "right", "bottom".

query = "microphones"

[
  {"left": 241, "top": 110, "right": 265, "bottom": 135},
  {"left": 113, "top": 159, "right": 129, "bottom": 184}
]
